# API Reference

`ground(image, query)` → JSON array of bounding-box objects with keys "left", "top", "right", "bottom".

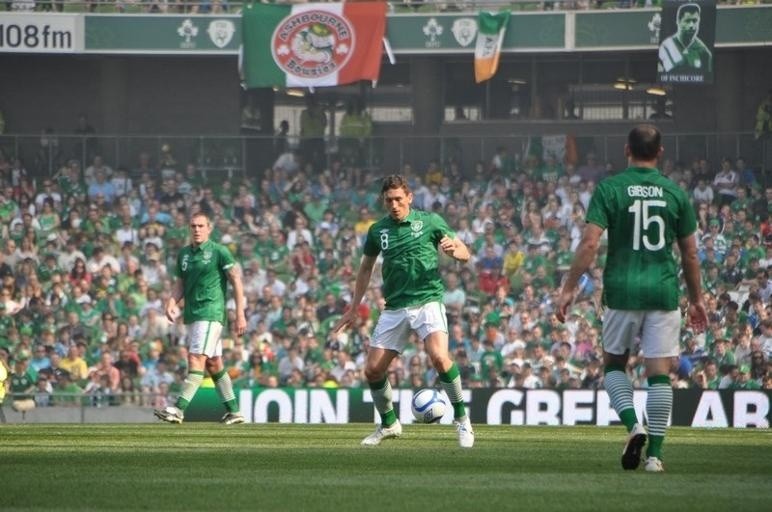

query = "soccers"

[{"left": 411, "top": 386, "right": 446, "bottom": 423}]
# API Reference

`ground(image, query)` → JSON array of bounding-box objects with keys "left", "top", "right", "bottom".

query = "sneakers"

[
  {"left": 154, "top": 407, "right": 184, "bottom": 424},
  {"left": 621, "top": 424, "right": 647, "bottom": 471},
  {"left": 455, "top": 417, "right": 474, "bottom": 448},
  {"left": 645, "top": 455, "right": 664, "bottom": 473},
  {"left": 361, "top": 420, "right": 402, "bottom": 445},
  {"left": 222, "top": 412, "right": 245, "bottom": 425}
]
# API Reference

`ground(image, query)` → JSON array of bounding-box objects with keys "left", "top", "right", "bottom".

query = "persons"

[
  {"left": 334, "top": 174, "right": 474, "bottom": 448},
  {"left": 156, "top": 94, "right": 401, "bottom": 386},
  {"left": 403, "top": 146, "right": 650, "bottom": 387},
  {"left": 2, "top": 111, "right": 187, "bottom": 409},
  {"left": 556, "top": 123, "right": 710, "bottom": 471},
  {"left": 657, "top": 4, "right": 712, "bottom": 78},
  {"left": 0, "top": 0, "right": 772, "bottom": 14},
  {"left": 153, "top": 211, "right": 246, "bottom": 425},
  {"left": 660, "top": 156, "right": 772, "bottom": 390}
]
